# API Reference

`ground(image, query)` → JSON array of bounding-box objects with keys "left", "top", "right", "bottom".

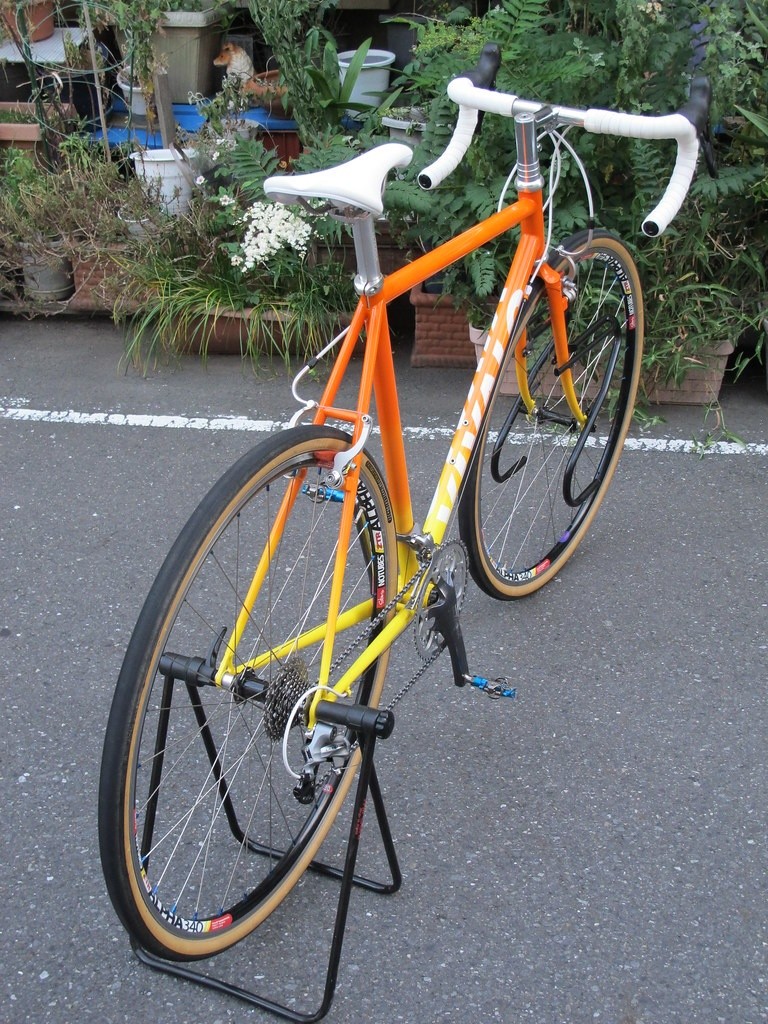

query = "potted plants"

[{"left": 0, "top": 0, "right": 768, "bottom": 406}]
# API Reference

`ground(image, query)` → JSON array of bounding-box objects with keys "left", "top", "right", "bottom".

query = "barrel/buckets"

[
  {"left": 129, "top": 149, "right": 199, "bottom": 216},
  {"left": 336, "top": 50, "right": 396, "bottom": 121},
  {"left": 18, "top": 229, "right": 80, "bottom": 301}
]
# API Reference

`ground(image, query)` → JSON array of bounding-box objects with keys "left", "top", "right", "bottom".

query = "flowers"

[{"left": 195, "top": 176, "right": 331, "bottom": 275}]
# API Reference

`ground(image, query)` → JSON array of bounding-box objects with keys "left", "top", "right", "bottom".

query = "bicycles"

[{"left": 97, "top": 43, "right": 714, "bottom": 1023}]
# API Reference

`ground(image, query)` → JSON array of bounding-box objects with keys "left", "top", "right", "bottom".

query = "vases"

[
  {"left": 333, "top": 50, "right": 397, "bottom": 107},
  {"left": 129, "top": 149, "right": 200, "bottom": 220},
  {"left": 243, "top": 69, "right": 293, "bottom": 113}
]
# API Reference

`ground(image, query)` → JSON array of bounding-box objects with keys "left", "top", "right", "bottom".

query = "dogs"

[{"left": 212, "top": 43, "right": 258, "bottom": 112}]
